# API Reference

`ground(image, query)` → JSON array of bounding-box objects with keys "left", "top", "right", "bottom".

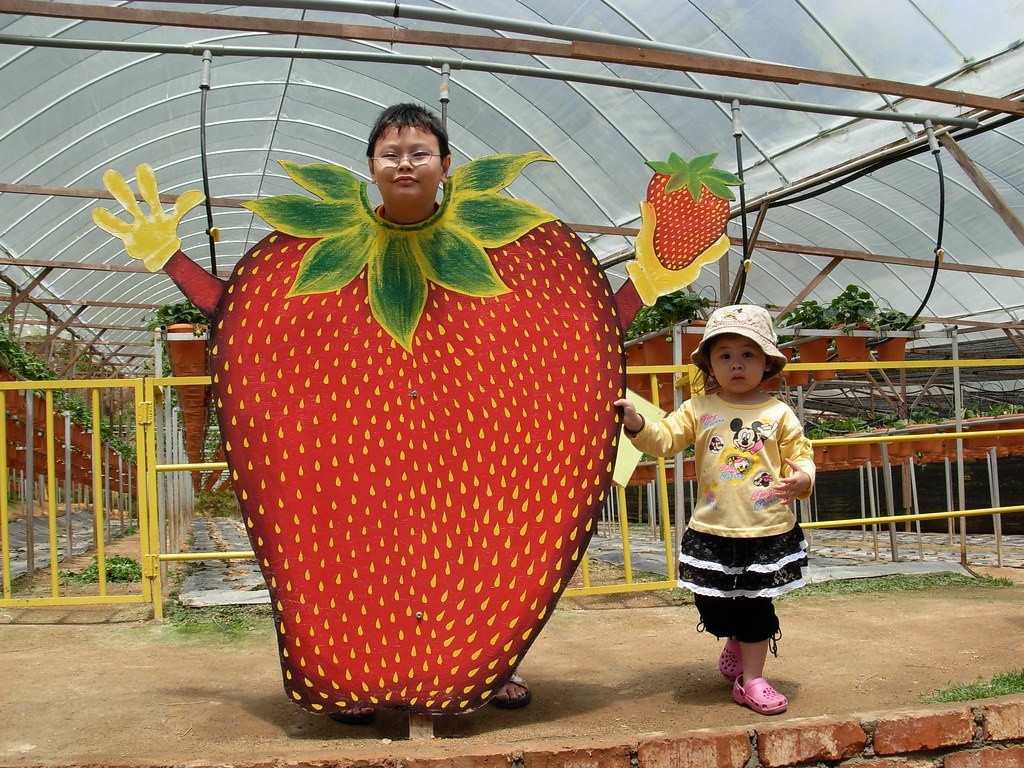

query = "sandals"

[
  {"left": 719, "top": 637, "right": 744, "bottom": 680},
  {"left": 733, "top": 673, "right": 788, "bottom": 715},
  {"left": 491, "top": 674, "right": 532, "bottom": 710},
  {"left": 329, "top": 709, "right": 374, "bottom": 726}
]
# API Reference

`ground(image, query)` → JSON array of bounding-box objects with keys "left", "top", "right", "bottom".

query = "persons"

[
  {"left": 613, "top": 305, "right": 816, "bottom": 715},
  {"left": 327, "top": 102, "right": 530, "bottom": 723}
]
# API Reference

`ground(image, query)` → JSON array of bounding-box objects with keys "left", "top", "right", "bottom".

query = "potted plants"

[
  {"left": 0, "top": 296, "right": 233, "bottom": 495},
  {"left": 611, "top": 283, "right": 1024, "bottom": 486}
]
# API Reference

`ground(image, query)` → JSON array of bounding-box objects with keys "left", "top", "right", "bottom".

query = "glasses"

[{"left": 371, "top": 146, "right": 444, "bottom": 168}]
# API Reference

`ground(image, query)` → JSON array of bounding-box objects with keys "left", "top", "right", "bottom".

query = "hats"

[{"left": 691, "top": 304, "right": 787, "bottom": 382}]
